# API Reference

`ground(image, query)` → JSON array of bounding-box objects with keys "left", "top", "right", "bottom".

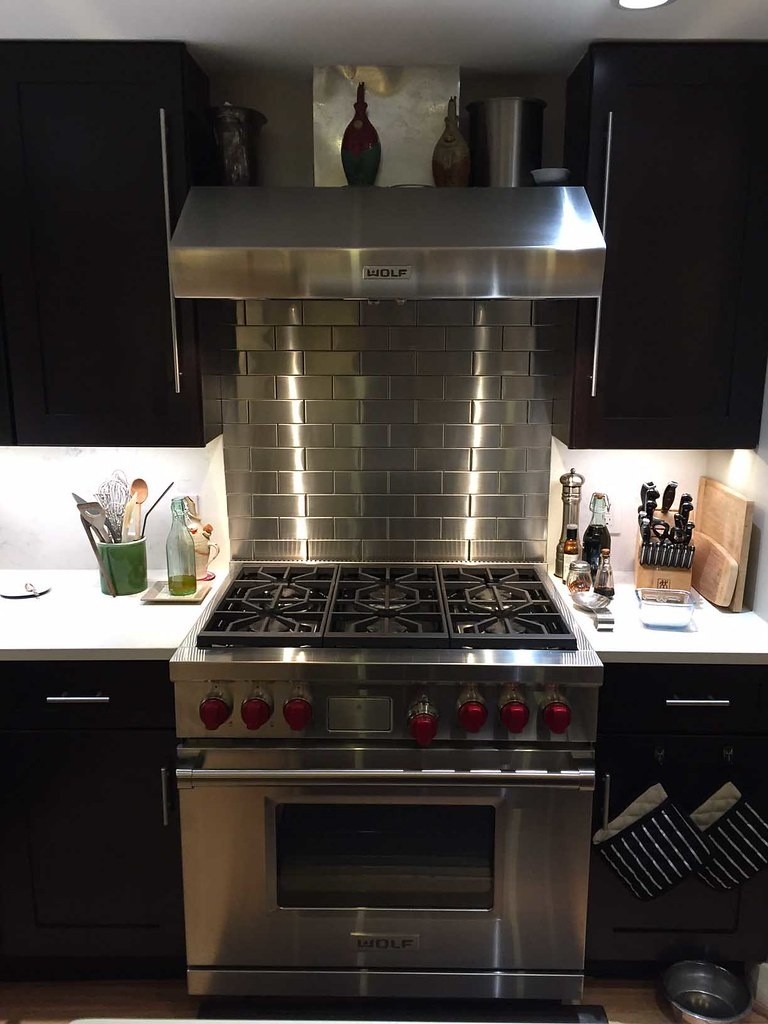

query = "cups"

[
  {"left": 465, "top": 97, "right": 549, "bottom": 187},
  {"left": 98, "top": 536, "right": 148, "bottom": 596}
]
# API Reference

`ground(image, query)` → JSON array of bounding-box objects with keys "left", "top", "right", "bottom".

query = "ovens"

[{"left": 174, "top": 743, "right": 597, "bottom": 1006}]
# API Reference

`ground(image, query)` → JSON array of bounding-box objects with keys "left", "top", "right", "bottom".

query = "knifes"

[
  {"left": 73, "top": 493, "right": 117, "bottom": 542},
  {"left": 638, "top": 480, "right": 696, "bottom": 569}
]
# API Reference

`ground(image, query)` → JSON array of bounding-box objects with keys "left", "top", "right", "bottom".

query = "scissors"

[{"left": 652, "top": 519, "right": 687, "bottom": 545}]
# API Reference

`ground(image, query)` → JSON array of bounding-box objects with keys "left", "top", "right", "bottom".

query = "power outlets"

[
  {"left": 179, "top": 494, "right": 199, "bottom": 521},
  {"left": 604, "top": 503, "right": 623, "bottom": 534}
]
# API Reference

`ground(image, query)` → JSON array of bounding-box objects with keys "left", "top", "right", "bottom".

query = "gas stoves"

[{"left": 170, "top": 559, "right": 603, "bottom": 743}]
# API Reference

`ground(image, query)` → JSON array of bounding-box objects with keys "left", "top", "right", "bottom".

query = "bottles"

[
  {"left": 594, "top": 548, "right": 616, "bottom": 600},
  {"left": 563, "top": 524, "right": 582, "bottom": 584},
  {"left": 340, "top": 81, "right": 381, "bottom": 185},
  {"left": 567, "top": 561, "right": 592, "bottom": 597},
  {"left": 431, "top": 96, "right": 471, "bottom": 188},
  {"left": 553, "top": 468, "right": 582, "bottom": 578},
  {"left": 583, "top": 492, "right": 613, "bottom": 585},
  {"left": 165, "top": 499, "right": 197, "bottom": 596}
]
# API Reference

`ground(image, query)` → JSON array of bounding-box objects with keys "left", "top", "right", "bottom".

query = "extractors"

[{"left": 171, "top": 63, "right": 607, "bottom": 298}]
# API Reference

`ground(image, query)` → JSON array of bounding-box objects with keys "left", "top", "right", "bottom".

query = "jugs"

[{"left": 184, "top": 496, "right": 219, "bottom": 580}]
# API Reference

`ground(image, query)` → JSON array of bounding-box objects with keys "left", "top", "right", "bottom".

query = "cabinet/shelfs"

[
  {"left": 0, "top": 39, "right": 223, "bottom": 447},
  {"left": 0, "top": 661, "right": 187, "bottom": 984},
  {"left": 552, "top": 39, "right": 768, "bottom": 451},
  {"left": 585, "top": 663, "right": 768, "bottom": 983}
]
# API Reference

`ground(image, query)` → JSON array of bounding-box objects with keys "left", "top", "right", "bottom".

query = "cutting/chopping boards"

[{"left": 696, "top": 475, "right": 755, "bottom": 613}]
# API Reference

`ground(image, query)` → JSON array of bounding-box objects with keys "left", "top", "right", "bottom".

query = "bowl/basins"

[
  {"left": 531, "top": 168, "right": 571, "bottom": 185},
  {"left": 654, "top": 961, "right": 755, "bottom": 1024},
  {"left": 635, "top": 587, "right": 696, "bottom": 628}
]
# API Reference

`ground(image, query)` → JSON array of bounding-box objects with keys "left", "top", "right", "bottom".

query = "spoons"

[
  {"left": 130, "top": 479, "right": 148, "bottom": 540},
  {"left": 25, "top": 584, "right": 39, "bottom": 596}
]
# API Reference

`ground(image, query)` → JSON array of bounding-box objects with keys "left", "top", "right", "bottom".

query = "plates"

[{"left": 0, "top": 579, "right": 52, "bottom": 597}]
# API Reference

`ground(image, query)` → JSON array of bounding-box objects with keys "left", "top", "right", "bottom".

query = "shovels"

[{"left": 77, "top": 501, "right": 115, "bottom": 544}]
